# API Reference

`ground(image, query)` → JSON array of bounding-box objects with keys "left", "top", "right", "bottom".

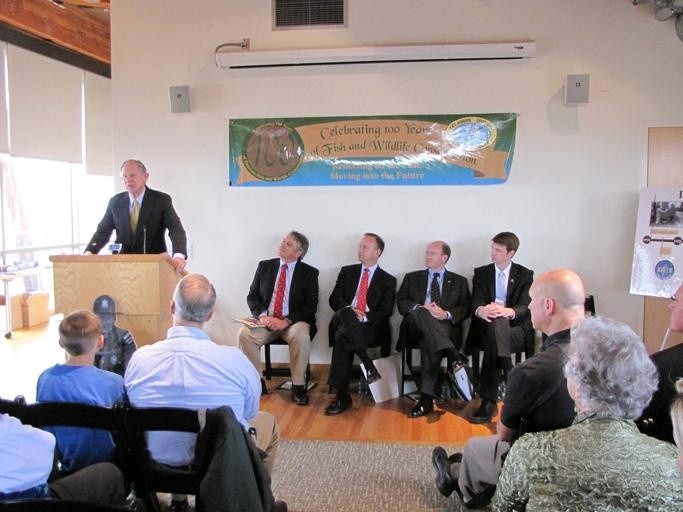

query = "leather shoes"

[
  {"left": 273, "top": 500, "right": 287, "bottom": 511},
  {"left": 451, "top": 355, "right": 463, "bottom": 373},
  {"left": 469, "top": 400, "right": 498, "bottom": 424},
  {"left": 432, "top": 446, "right": 462, "bottom": 498},
  {"left": 367, "top": 368, "right": 381, "bottom": 384},
  {"left": 169, "top": 499, "right": 190, "bottom": 512},
  {"left": 325, "top": 394, "right": 352, "bottom": 414},
  {"left": 409, "top": 400, "right": 434, "bottom": 418},
  {"left": 292, "top": 388, "right": 308, "bottom": 406}
]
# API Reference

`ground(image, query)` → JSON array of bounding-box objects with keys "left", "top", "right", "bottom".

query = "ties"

[
  {"left": 357, "top": 268, "right": 368, "bottom": 313},
  {"left": 430, "top": 273, "right": 441, "bottom": 308},
  {"left": 130, "top": 200, "right": 139, "bottom": 234},
  {"left": 495, "top": 272, "right": 506, "bottom": 300},
  {"left": 273, "top": 264, "right": 287, "bottom": 318}
]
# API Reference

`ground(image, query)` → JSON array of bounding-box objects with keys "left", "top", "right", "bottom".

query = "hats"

[{"left": 94, "top": 295, "right": 115, "bottom": 315}]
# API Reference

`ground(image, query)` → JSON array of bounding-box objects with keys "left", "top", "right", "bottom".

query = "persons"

[
  {"left": 488, "top": 311, "right": 683, "bottom": 511},
  {"left": 635, "top": 282, "right": 682, "bottom": 444},
  {"left": 80, "top": 160, "right": 187, "bottom": 271},
  {"left": 0, "top": 411, "right": 126, "bottom": 512},
  {"left": 655, "top": 205, "right": 668, "bottom": 224},
  {"left": 89, "top": 291, "right": 136, "bottom": 374},
  {"left": 34, "top": 308, "right": 130, "bottom": 475},
  {"left": 324, "top": 232, "right": 396, "bottom": 415},
  {"left": 124, "top": 272, "right": 288, "bottom": 511},
  {"left": 396, "top": 241, "right": 471, "bottom": 418},
  {"left": 669, "top": 376, "right": 682, "bottom": 475},
  {"left": 237, "top": 229, "right": 318, "bottom": 405},
  {"left": 431, "top": 267, "right": 586, "bottom": 511},
  {"left": 463, "top": 231, "right": 534, "bottom": 424}
]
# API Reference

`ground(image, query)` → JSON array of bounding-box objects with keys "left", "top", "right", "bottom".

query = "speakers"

[
  {"left": 169, "top": 84, "right": 191, "bottom": 114},
  {"left": 563, "top": 73, "right": 591, "bottom": 107}
]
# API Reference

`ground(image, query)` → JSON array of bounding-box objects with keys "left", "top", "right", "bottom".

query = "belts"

[{"left": 164, "top": 463, "right": 191, "bottom": 472}]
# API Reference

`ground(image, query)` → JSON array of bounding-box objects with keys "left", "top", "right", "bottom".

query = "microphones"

[
  {"left": 108, "top": 238, "right": 124, "bottom": 255},
  {"left": 143, "top": 222, "right": 147, "bottom": 254}
]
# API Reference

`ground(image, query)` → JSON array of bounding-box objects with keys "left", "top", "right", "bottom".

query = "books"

[
  {"left": 232, "top": 315, "right": 266, "bottom": 328},
  {"left": 448, "top": 364, "right": 475, "bottom": 402}
]
